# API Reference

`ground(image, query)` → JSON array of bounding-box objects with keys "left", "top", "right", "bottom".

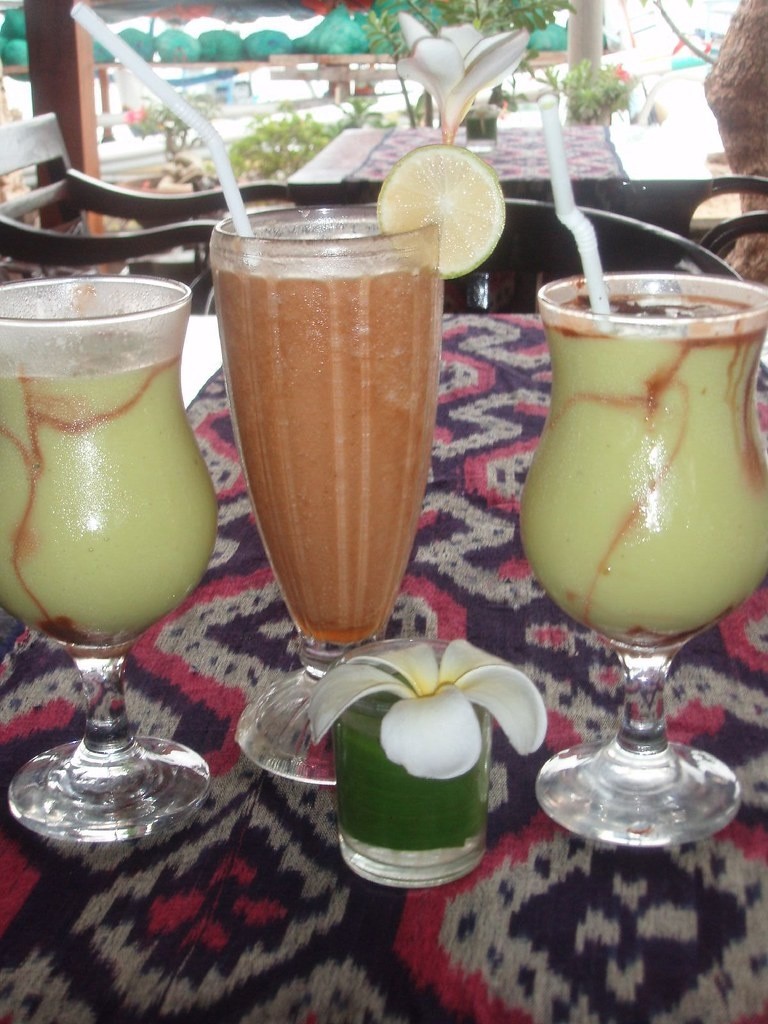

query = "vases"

[
  {"left": 324, "top": 638, "right": 493, "bottom": 887},
  {"left": 466, "top": 117, "right": 497, "bottom": 153}
]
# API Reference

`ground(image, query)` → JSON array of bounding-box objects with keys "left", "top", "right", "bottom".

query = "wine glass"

[
  {"left": 516, "top": 270, "right": 765, "bottom": 849},
  {"left": 208, "top": 205, "right": 443, "bottom": 785},
  {"left": 0, "top": 275, "right": 218, "bottom": 847}
]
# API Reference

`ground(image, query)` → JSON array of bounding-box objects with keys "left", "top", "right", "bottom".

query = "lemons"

[{"left": 376, "top": 143, "right": 507, "bottom": 281}]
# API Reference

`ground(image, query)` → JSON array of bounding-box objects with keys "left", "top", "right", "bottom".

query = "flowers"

[
  {"left": 394, "top": 10, "right": 529, "bottom": 144},
  {"left": 296, "top": 636, "right": 549, "bottom": 782},
  {"left": 466, "top": 89, "right": 502, "bottom": 135}
]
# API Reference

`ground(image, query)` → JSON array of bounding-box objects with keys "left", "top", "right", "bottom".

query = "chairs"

[{"left": 0, "top": 112, "right": 292, "bottom": 323}]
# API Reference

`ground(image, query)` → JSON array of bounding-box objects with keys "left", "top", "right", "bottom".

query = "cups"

[
  {"left": 326, "top": 639, "right": 493, "bottom": 890},
  {"left": 464, "top": 104, "right": 498, "bottom": 153}
]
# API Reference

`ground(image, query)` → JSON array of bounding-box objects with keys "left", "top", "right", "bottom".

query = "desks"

[
  {"left": 287, "top": 122, "right": 729, "bottom": 231},
  {"left": 0, "top": 311, "right": 768, "bottom": 1024}
]
